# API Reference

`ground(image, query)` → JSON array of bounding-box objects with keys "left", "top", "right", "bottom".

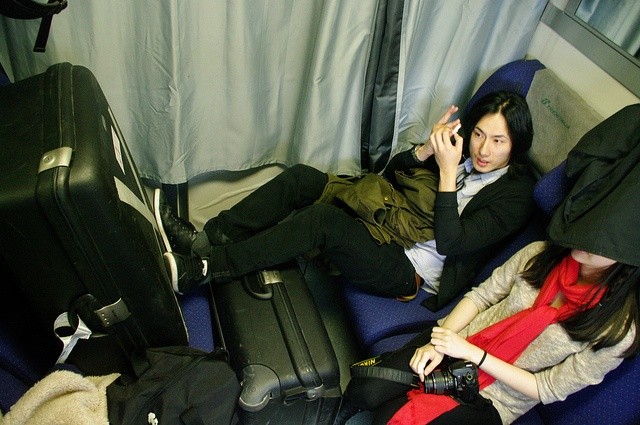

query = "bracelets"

[
  {"left": 411, "top": 144, "right": 425, "bottom": 162},
  {"left": 476, "top": 350, "right": 487, "bottom": 367}
]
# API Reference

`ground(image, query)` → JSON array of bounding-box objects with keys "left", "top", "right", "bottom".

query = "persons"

[
  {"left": 153, "top": 90, "right": 537, "bottom": 313},
  {"left": 341, "top": 236, "right": 636, "bottom": 425}
]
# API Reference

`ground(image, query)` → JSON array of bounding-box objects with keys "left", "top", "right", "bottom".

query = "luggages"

[
  {"left": 208, "top": 260, "right": 344, "bottom": 425},
  {"left": 0, "top": 61, "right": 188, "bottom": 374}
]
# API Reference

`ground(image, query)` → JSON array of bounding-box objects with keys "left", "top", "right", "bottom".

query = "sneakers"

[
  {"left": 148, "top": 187, "right": 196, "bottom": 256},
  {"left": 164, "top": 250, "right": 204, "bottom": 297}
]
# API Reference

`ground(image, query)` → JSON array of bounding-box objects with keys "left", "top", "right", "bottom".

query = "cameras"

[{"left": 424, "top": 359, "right": 480, "bottom": 406}]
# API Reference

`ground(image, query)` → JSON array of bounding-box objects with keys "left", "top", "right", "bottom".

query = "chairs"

[
  {"left": 370, "top": 332, "right": 639, "bottom": 425},
  {"left": 0, "top": 291, "right": 214, "bottom": 387},
  {"left": 340, "top": 57, "right": 640, "bottom": 350}
]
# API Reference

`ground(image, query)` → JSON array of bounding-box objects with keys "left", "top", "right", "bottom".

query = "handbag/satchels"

[{"left": 107, "top": 345, "right": 240, "bottom": 424}]
